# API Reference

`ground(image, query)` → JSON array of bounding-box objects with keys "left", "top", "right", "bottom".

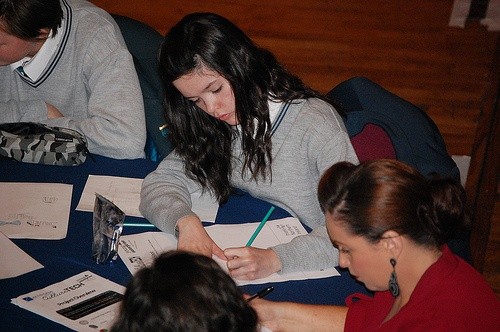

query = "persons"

[
  {"left": 0, "top": 0, "right": 147, "bottom": 159},
  {"left": 109, "top": 250, "right": 260, "bottom": 332},
  {"left": 139, "top": 12, "right": 361, "bottom": 281},
  {"left": 243, "top": 158, "right": 500, "bottom": 332}
]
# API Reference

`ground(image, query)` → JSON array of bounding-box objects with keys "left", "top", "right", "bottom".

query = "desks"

[{"left": 0, "top": 149, "right": 377, "bottom": 332}]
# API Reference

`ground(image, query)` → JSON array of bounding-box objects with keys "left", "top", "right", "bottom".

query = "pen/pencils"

[
  {"left": 245, "top": 286, "right": 274, "bottom": 303},
  {"left": 244, "top": 206, "right": 276, "bottom": 247},
  {"left": 116, "top": 223, "right": 155, "bottom": 227}
]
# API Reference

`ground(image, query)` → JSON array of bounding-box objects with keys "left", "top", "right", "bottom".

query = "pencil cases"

[{"left": 0, "top": 122, "right": 88, "bottom": 165}]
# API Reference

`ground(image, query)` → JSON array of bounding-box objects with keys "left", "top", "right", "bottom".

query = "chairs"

[
  {"left": 111, "top": 13, "right": 172, "bottom": 157},
  {"left": 324, "top": 76, "right": 461, "bottom": 182}
]
